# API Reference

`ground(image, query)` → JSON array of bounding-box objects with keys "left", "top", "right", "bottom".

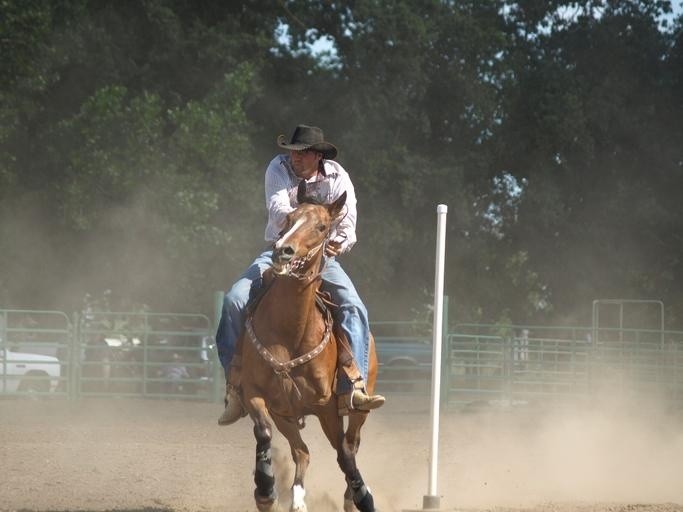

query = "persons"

[
  {"left": 47, "top": 316, "right": 216, "bottom": 395},
  {"left": 213, "top": 123, "right": 386, "bottom": 426}
]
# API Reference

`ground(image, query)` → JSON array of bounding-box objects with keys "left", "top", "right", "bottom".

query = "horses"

[{"left": 238, "top": 179, "right": 377, "bottom": 512}]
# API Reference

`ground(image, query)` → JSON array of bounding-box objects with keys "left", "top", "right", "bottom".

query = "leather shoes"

[
  {"left": 217, "top": 392, "right": 247, "bottom": 424},
  {"left": 338, "top": 390, "right": 385, "bottom": 416}
]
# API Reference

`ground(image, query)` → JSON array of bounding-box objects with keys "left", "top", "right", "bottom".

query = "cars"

[{"left": 0, "top": 350, "right": 61, "bottom": 395}]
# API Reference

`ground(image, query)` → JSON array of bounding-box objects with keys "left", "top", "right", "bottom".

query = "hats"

[{"left": 277, "top": 125, "right": 337, "bottom": 160}]
